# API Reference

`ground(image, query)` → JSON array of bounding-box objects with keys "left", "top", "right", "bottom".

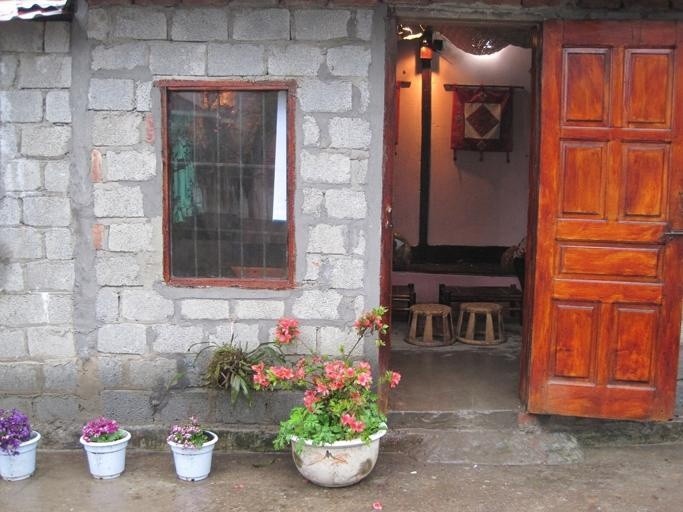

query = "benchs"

[{"left": 436, "top": 284, "right": 524, "bottom": 325}]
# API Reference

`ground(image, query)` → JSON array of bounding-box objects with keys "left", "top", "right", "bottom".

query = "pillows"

[
  {"left": 515, "top": 237, "right": 527, "bottom": 259},
  {"left": 502, "top": 245, "right": 514, "bottom": 267},
  {"left": 395, "top": 234, "right": 412, "bottom": 267}
]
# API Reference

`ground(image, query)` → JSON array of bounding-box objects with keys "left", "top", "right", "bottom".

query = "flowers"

[
  {"left": 79, "top": 418, "right": 130, "bottom": 445},
  {"left": 167, "top": 415, "right": 218, "bottom": 452},
  {"left": 194, "top": 307, "right": 402, "bottom": 452},
  {"left": 0, "top": 413, "right": 41, "bottom": 451}
]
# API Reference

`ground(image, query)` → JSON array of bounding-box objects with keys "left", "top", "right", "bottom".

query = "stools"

[
  {"left": 456, "top": 302, "right": 508, "bottom": 345},
  {"left": 405, "top": 300, "right": 454, "bottom": 347},
  {"left": 392, "top": 281, "right": 419, "bottom": 321}
]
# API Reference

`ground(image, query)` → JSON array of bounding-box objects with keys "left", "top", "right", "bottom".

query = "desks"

[{"left": 392, "top": 257, "right": 521, "bottom": 305}]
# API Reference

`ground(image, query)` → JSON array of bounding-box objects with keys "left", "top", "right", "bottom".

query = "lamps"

[{"left": 417, "top": 39, "right": 433, "bottom": 69}]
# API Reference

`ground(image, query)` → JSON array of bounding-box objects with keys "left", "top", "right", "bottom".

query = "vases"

[
  {"left": 288, "top": 421, "right": 387, "bottom": 489},
  {"left": 0, "top": 426, "right": 38, "bottom": 489},
  {"left": 79, "top": 432, "right": 131, "bottom": 482},
  {"left": 167, "top": 434, "right": 216, "bottom": 484}
]
